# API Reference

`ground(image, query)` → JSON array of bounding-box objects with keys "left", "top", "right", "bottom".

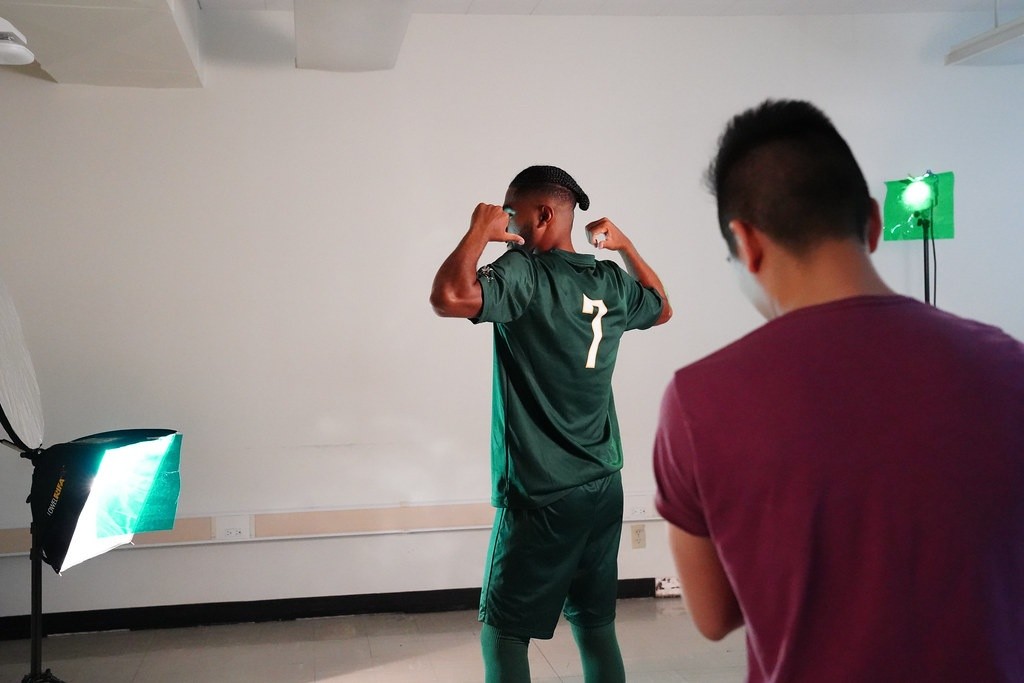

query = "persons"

[
  {"left": 429, "top": 167, "right": 673, "bottom": 683},
  {"left": 652, "top": 99, "right": 1024, "bottom": 682}
]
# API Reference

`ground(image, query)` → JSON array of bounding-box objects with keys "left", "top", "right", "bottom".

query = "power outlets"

[{"left": 210, "top": 513, "right": 256, "bottom": 541}]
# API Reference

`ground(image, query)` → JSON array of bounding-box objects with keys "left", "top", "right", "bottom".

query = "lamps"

[
  {"left": 882, "top": 168, "right": 955, "bottom": 310},
  {"left": 1, "top": 428, "right": 184, "bottom": 683}
]
